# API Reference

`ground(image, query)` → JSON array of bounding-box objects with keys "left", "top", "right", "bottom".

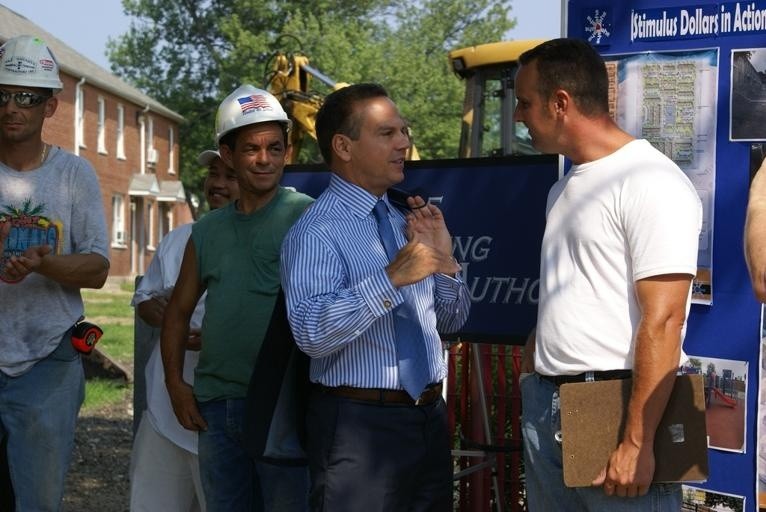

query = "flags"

[{"left": 238, "top": 94, "right": 270, "bottom": 112}]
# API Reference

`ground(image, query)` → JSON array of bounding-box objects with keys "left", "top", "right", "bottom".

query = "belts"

[
  {"left": 325, "top": 382, "right": 443, "bottom": 406},
  {"left": 536, "top": 371, "right": 635, "bottom": 386}
]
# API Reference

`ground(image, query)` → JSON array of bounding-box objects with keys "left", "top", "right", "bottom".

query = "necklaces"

[{"left": 40, "top": 142, "right": 46, "bottom": 164}]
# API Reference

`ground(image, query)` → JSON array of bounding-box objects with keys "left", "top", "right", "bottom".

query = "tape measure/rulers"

[{"left": 71, "top": 322, "right": 103, "bottom": 355}]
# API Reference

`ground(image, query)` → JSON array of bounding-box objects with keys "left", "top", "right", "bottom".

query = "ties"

[{"left": 372, "top": 200, "right": 430, "bottom": 400}]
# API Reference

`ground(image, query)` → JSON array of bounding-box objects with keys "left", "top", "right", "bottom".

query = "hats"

[{"left": 195, "top": 150, "right": 221, "bottom": 166}]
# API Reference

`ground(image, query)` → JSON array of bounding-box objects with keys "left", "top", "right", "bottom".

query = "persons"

[
  {"left": 0, "top": 33, "right": 110, "bottom": 511},
  {"left": 743, "top": 158, "right": 766, "bottom": 304},
  {"left": 159, "top": 85, "right": 316, "bottom": 512},
  {"left": 127, "top": 150, "right": 239, "bottom": 511},
  {"left": 511, "top": 38, "right": 702, "bottom": 511},
  {"left": 280, "top": 83, "right": 471, "bottom": 512}
]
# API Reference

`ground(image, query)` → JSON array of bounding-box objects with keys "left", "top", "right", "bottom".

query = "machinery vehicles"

[{"left": 249, "top": 32, "right": 548, "bottom": 163}]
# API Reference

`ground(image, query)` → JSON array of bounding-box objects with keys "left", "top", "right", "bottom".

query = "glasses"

[{"left": 2, "top": 89, "right": 53, "bottom": 109}]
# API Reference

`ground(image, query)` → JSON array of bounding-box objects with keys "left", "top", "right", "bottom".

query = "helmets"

[
  {"left": 215, "top": 83, "right": 291, "bottom": 147},
  {"left": 0, "top": 35, "right": 63, "bottom": 94}
]
